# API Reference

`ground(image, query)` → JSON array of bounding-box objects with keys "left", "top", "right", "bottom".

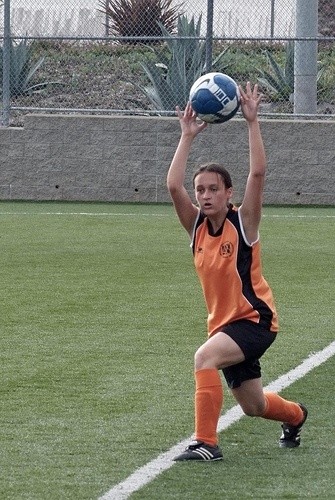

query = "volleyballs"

[{"left": 188, "top": 71, "right": 242, "bottom": 125}]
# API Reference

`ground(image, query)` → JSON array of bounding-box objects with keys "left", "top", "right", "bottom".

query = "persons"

[{"left": 167, "top": 82, "right": 308, "bottom": 462}]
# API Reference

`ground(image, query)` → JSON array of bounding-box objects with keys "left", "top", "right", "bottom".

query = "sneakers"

[
  {"left": 172, "top": 439, "right": 224, "bottom": 463},
  {"left": 278, "top": 402, "right": 308, "bottom": 449}
]
